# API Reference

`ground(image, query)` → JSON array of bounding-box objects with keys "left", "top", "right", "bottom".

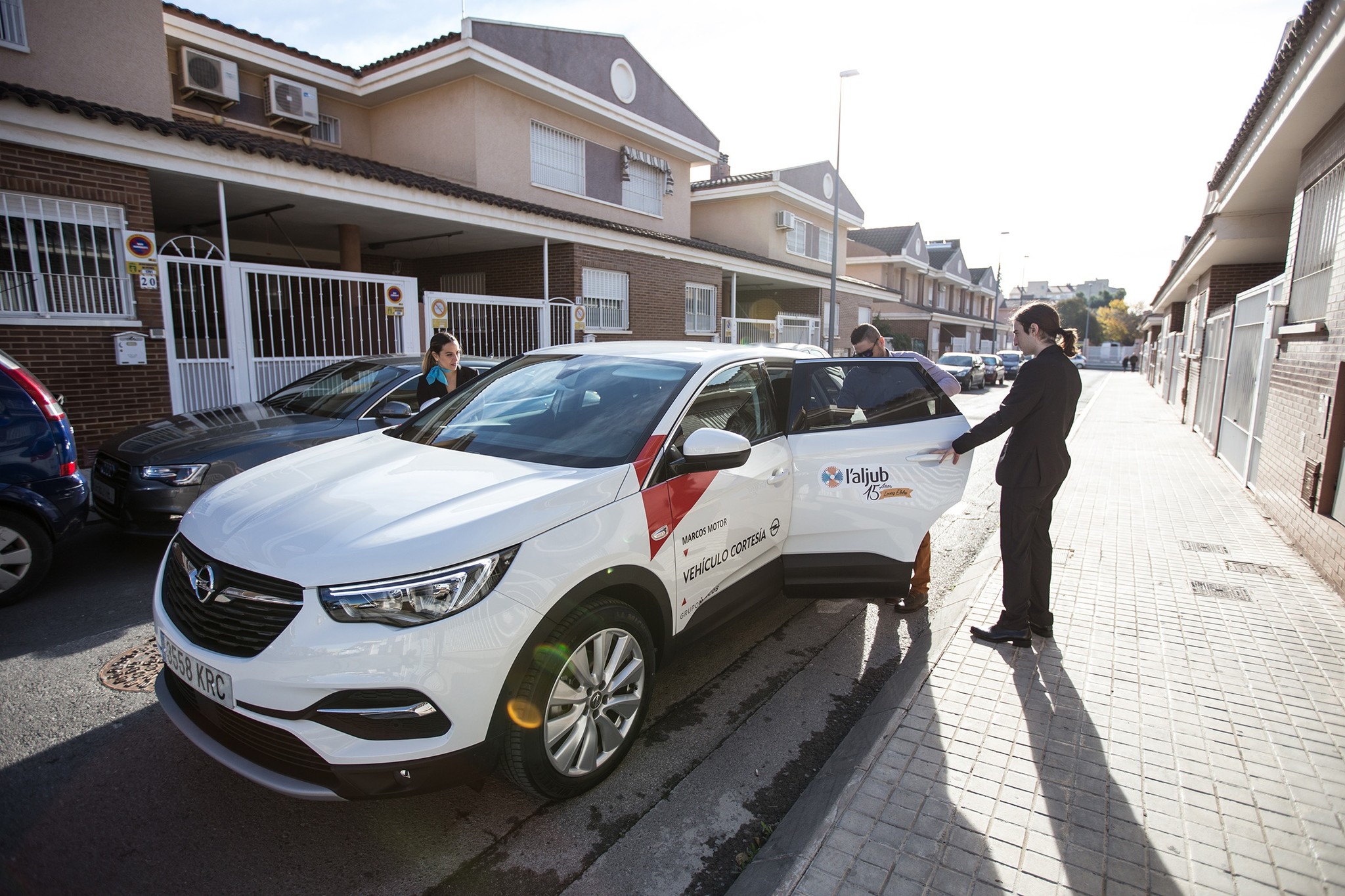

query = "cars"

[
  {"left": 1067, "top": 354, "right": 1088, "bottom": 369},
  {"left": 90, "top": 353, "right": 603, "bottom": 547},
  {"left": 935, "top": 351, "right": 986, "bottom": 391},
  {"left": 976, "top": 354, "right": 1005, "bottom": 385},
  {"left": 995, "top": 350, "right": 1025, "bottom": 381}
]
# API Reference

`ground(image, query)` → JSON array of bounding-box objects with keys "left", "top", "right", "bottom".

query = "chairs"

[{"left": 771, "top": 378, "right": 811, "bottom": 432}]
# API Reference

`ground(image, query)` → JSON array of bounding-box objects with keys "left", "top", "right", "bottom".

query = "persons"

[
  {"left": 1122, "top": 354, "right": 1128, "bottom": 372},
  {"left": 415, "top": 333, "right": 486, "bottom": 426},
  {"left": 1129, "top": 352, "right": 1138, "bottom": 372},
  {"left": 833, "top": 324, "right": 961, "bottom": 613},
  {"left": 928, "top": 302, "right": 1083, "bottom": 649}
]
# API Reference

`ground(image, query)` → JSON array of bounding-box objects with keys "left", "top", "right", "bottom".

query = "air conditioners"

[
  {"left": 263, "top": 73, "right": 319, "bottom": 126},
  {"left": 776, "top": 210, "right": 795, "bottom": 229},
  {"left": 177, "top": 45, "right": 241, "bottom": 104}
]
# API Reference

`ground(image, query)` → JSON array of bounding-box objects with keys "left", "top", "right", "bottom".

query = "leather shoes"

[
  {"left": 971, "top": 624, "right": 1033, "bottom": 648},
  {"left": 1029, "top": 620, "right": 1053, "bottom": 637}
]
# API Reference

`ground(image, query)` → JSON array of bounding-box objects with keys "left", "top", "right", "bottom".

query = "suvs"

[
  {"left": 745, "top": 341, "right": 849, "bottom": 392},
  {"left": 0, "top": 348, "right": 88, "bottom": 608},
  {"left": 150, "top": 337, "right": 974, "bottom": 809}
]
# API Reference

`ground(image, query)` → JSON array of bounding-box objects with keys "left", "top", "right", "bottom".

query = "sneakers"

[
  {"left": 895, "top": 590, "right": 929, "bottom": 614},
  {"left": 886, "top": 597, "right": 901, "bottom": 606}
]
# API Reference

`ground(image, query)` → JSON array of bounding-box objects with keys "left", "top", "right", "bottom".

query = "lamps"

[{"left": 392, "top": 259, "right": 402, "bottom": 275}]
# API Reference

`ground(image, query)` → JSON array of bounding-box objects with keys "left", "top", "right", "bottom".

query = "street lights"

[
  {"left": 991, "top": 232, "right": 1031, "bottom": 355},
  {"left": 827, "top": 69, "right": 860, "bottom": 357},
  {"left": 1082, "top": 283, "right": 1093, "bottom": 355}
]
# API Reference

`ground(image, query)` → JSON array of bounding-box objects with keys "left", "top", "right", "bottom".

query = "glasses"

[{"left": 852, "top": 338, "right": 879, "bottom": 358}]
